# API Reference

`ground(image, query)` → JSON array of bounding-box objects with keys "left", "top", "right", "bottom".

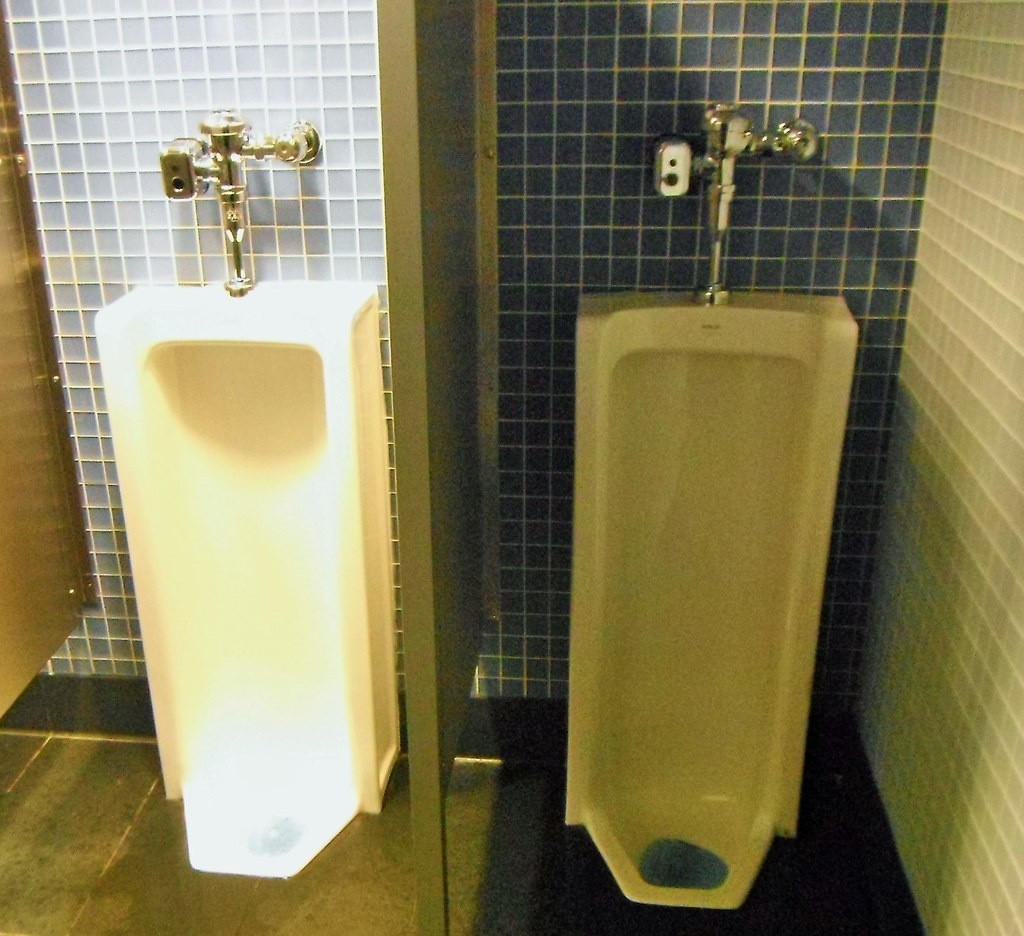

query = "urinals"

[
  {"left": 564, "top": 298, "right": 859, "bottom": 914},
  {"left": 90, "top": 278, "right": 399, "bottom": 886}
]
